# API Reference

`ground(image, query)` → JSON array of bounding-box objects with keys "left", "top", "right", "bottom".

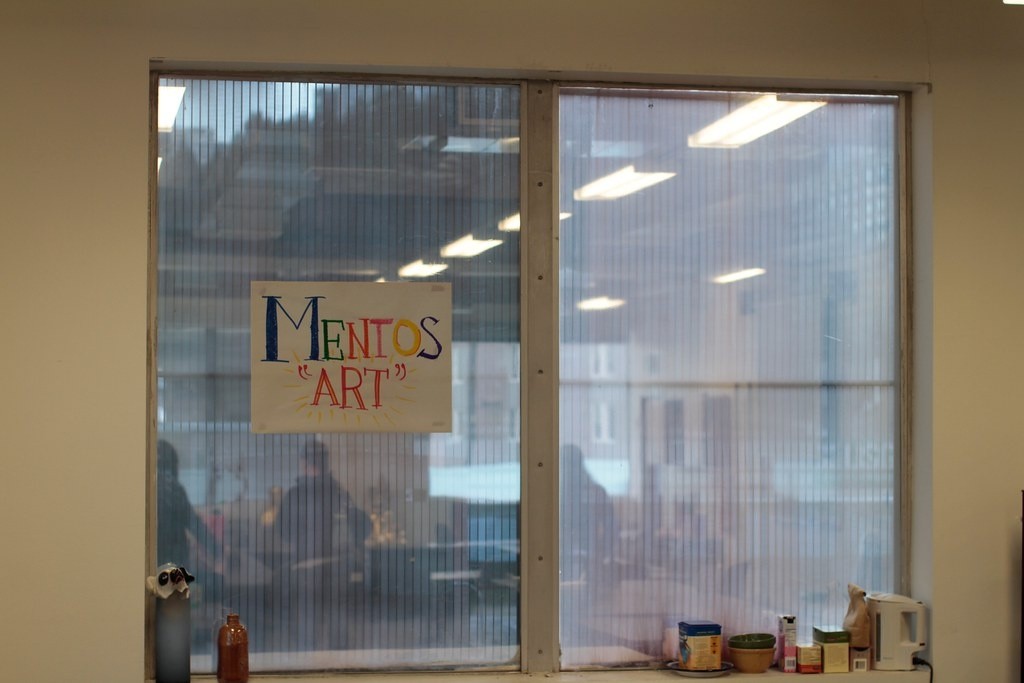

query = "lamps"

[
  {"left": 685, "top": 92, "right": 833, "bottom": 151},
  {"left": 397, "top": 209, "right": 571, "bottom": 281},
  {"left": 570, "top": 97, "right": 680, "bottom": 203}
]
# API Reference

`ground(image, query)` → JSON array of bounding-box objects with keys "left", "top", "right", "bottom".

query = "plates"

[{"left": 665, "top": 662, "right": 734, "bottom": 678}]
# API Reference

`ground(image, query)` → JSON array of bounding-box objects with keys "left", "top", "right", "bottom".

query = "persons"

[
  {"left": 262, "top": 438, "right": 373, "bottom": 649},
  {"left": 157, "top": 438, "right": 239, "bottom": 592},
  {"left": 561, "top": 444, "right": 614, "bottom": 600}
]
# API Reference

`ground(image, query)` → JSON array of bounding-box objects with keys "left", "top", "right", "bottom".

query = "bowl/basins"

[{"left": 728, "top": 632, "right": 776, "bottom": 673}]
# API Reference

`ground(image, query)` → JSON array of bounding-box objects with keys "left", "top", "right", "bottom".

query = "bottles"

[
  {"left": 153, "top": 563, "right": 192, "bottom": 683},
  {"left": 662, "top": 628, "right": 680, "bottom": 659},
  {"left": 217, "top": 613, "right": 250, "bottom": 683}
]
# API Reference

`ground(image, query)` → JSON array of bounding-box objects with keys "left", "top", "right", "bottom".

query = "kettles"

[{"left": 867, "top": 591, "right": 927, "bottom": 671}]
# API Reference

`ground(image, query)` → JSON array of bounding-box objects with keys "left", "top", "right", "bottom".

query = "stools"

[{"left": 430, "top": 571, "right": 484, "bottom": 646}]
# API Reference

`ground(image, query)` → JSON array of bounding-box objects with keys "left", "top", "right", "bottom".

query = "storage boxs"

[
  {"left": 796, "top": 644, "right": 822, "bottom": 673},
  {"left": 812, "top": 624, "right": 850, "bottom": 672},
  {"left": 677, "top": 620, "right": 721, "bottom": 671},
  {"left": 777, "top": 614, "right": 797, "bottom": 673}
]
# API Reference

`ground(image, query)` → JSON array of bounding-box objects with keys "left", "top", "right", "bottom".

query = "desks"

[{"left": 558, "top": 580, "right": 801, "bottom": 651}]
